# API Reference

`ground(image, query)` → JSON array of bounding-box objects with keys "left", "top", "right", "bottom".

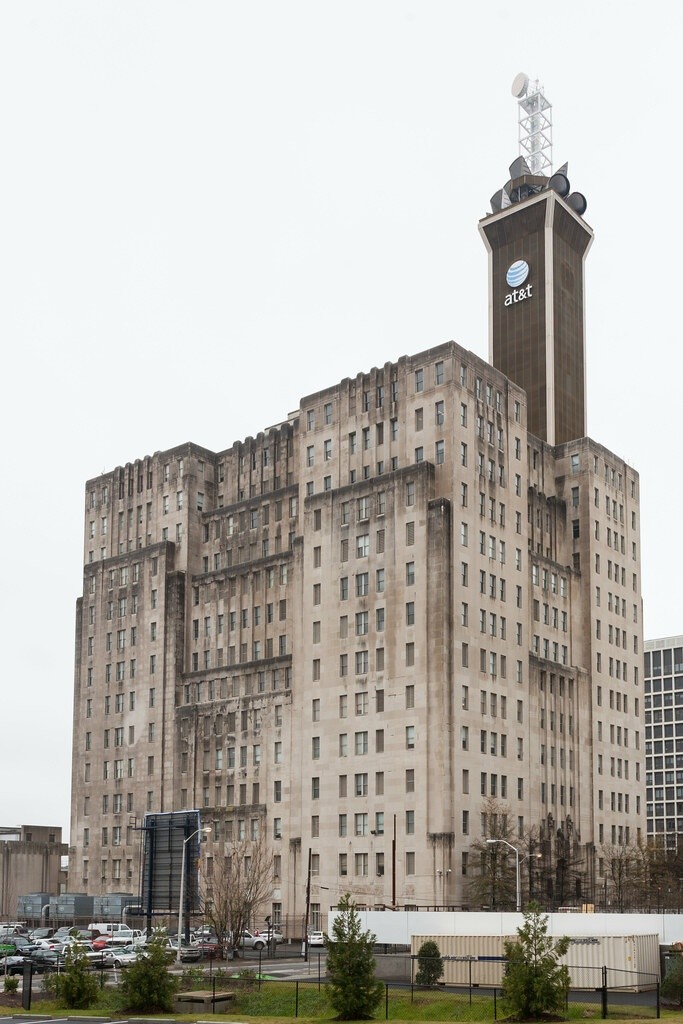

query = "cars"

[
  {"left": 0, "top": 923, "right": 267, "bottom": 973},
  {"left": 307, "top": 931, "right": 325, "bottom": 944},
  {"left": 259, "top": 930, "right": 284, "bottom": 944}
]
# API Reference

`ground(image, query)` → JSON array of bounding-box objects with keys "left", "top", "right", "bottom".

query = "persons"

[{"left": 254, "top": 927, "right": 259, "bottom": 937}]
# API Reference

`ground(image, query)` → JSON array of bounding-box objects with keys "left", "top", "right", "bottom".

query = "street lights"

[
  {"left": 487, "top": 839, "right": 542, "bottom": 912},
  {"left": 174, "top": 828, "right": 212, "bottom": 970},
  {"left": 436, "top": 868, "right": 451, "bottom": 911}
]
